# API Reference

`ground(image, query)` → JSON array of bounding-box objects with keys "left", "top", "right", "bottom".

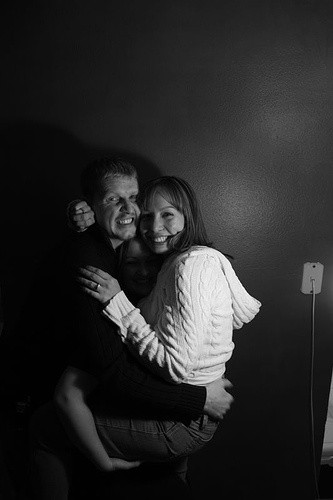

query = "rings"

[
  {"left": 80, "top": 208, "right": 85, "bottom": 214},
  {"left": 95, "top": 284, "right": 101, "bottom": 292}
]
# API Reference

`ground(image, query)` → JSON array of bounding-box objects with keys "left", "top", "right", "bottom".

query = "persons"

[
  {"left": 53, "top": 229, "right": 190, "bottom": 500},
  {"left": 0, "top": 155, "right": 235, "bottom": 500},
  {"left": 28, "top": 173, "right": 264, "bottom": 500}
]
